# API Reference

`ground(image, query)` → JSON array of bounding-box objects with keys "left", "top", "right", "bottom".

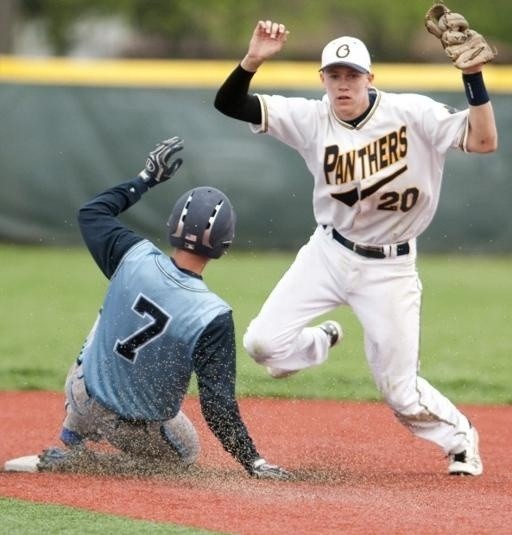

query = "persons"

[
  {"left": 36, "top": 135, "right": 296, "bottom": 480},
  {"left": 213, "top": 0, "right": 498, "bottom": 477}
]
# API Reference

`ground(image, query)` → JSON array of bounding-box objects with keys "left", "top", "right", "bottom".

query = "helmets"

[{"left": 167, "top": 187, "right": 235, "bottom": 259}]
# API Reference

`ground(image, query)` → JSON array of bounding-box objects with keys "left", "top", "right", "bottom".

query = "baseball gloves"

[{"left": 424, "top": 3, "right": 497, "bottom": 69}]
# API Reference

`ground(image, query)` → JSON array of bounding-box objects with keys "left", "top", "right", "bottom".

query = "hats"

[{"left": 318, "top": 36, "right": 371, "bottom": 73}]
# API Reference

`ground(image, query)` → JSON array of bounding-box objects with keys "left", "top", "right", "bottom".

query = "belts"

[
  {"left": 83, "top": 379, "right": 146, "bottom": 428},
  {"left": 322, "top": 223, "right": 410, "bottom": 258}
]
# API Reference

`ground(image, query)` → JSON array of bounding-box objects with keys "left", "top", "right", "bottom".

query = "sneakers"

[
  {"left": 58, "top": 428, "right": 84, "bottom": 450},
  {"left": 447, "top": 424, "right": 483, "bottom": 476},
  {"left": 38, "top": 445, "right": 96, "bottom": 473},
  {"left": 267, "top": 320, "right": 342, "bottom": 379}
]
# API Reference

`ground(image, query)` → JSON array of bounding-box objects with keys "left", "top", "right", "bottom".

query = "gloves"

[
  {"left": 138, "top": 137, "right": 185, "bottom": 188},
  {"left": 244, "top": 455, "right": 296, "bottom": 481}
]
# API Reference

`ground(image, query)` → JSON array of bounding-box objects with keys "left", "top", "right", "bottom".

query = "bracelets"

[{"left": 463, "top": 71, "right": 491, "bottom": 107}]
